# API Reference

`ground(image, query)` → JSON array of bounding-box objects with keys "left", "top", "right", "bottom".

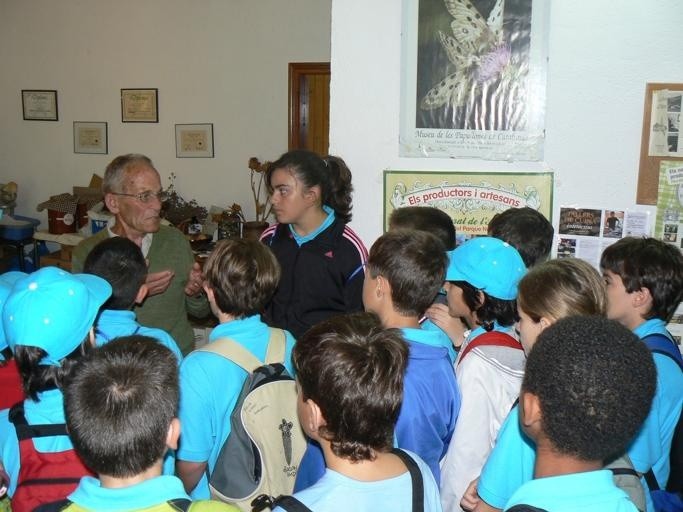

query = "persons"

[
  {"left": 83, "top": 236, "right": 184, "bottom": 367},
  {"left": 258, "top": 150, "right": 371, "bottom": 340},
  {"left": 71, "top": 152, "right": 210, "bottom": 359},
  {"left": 487, "top": 206, "right": 554, "bottom": 270},
  {"left": 605, "top": 212, "right": 620, "bottom": 232},
  {"left": 506, "top": 314, "right": 655, "bottom": 512},
  {"left": 31, "top": 336, "right": 242, "bottom": 511},
  {"left": 176, "top": 237, "right": 309, "bottom": 512},
  {"left": 0, "top": 270, "right": 38, "bottom": 413},
  {"left": 390, "top": 206, "right": 464, "bottom": 351},
  {"left": 252, "top": 312, "right": 442, "bottom": 512},
  {"left": 294, "top": 228, "right": 461, "bottom": 493},
  {"left": 0, "top": 266, "right": 111, "bottom": 512},
  {"left": 601, "top": 236, "right": 683, "bottom": 512},
  {"left": 459, "top": 253, "right": 656, "bottom": 512},
  {"left": 438, "top": 236, "right": 528, "bottom": 512}
]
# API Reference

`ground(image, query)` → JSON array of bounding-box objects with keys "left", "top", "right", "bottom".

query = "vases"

[
  {"left": 218, "top": 220, "right": 241, "bottom": 241},
  {"left": 243, "top": 222, "right": 270, "bottom": 241}
]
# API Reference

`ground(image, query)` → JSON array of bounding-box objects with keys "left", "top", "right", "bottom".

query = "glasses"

[{"left": 109, "top": 189, "right": 162, "bottom": 202}]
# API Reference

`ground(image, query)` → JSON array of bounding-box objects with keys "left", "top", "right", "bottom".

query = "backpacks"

[
  {"left": 10, "top": 398, "right": 100, "bottom": 512},
  {"left": 640, "top": 329, "right": 683, "bottom": 512},
  {"left": 188, "top": 325, "right": 309, "bottom": 512}
]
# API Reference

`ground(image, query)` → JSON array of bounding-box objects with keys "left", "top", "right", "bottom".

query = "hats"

[
  {"left": 0, "top": 270, "right": 27, "bottom": 362},
  {"left": 3, "top": 265, "right": 113, "bottom": 369},
  {"left": 443, "top": 235, "right": 528, "bottom": 302}
]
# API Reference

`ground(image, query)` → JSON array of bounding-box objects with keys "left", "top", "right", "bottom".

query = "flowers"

[
  {"left": 231, "top": 156, "right": 273, "bottom": 223},
  {"left": 210, "top": 204, "right": 245, "bottom": 223}
]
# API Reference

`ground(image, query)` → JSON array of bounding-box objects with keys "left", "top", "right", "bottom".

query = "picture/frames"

[
  {"left": 175, "top": 123, "right": 214, "bottom": 158},
  {"left": 73, "top": 121, "right": 108, "bottom": 155},
  {"left": 120, "top": 88, "right": 159, "bottom": 123},
  {"left": 21, "top": 88, "right": 59, "bottom": 121}
]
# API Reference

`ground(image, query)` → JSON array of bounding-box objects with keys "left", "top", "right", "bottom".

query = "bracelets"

[{"left": 452, "top": 344, "right": 462, "bottom": 353}]
